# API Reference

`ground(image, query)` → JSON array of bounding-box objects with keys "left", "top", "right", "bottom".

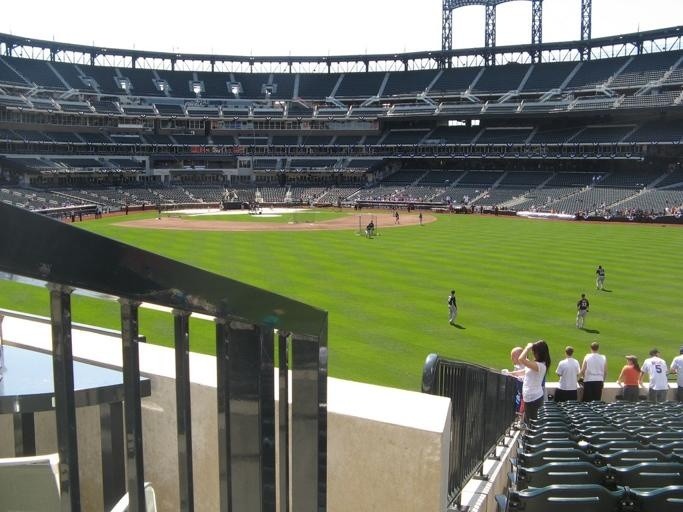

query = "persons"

[
  {"left": 639, "top": 348, "right": 669, "bottom": 405},
  {"left": 595, "top": 263, "right": 605, "bottom": 291},
  {"left": 668, "top": 347, "right": 682, "bottom": 402},
  {"left": 507, "top": 339, "right": 552, "bottom": 423},
  {"left": 574, "top": 292, "right": 589, "bottom": 328},
  {"left": 616, "top": 354, "right": 641, "bottom": 402},
  {"left": 446, "top": 287, "right": 457, "bottom": 325},
  {"left": 509, "top": 345, "right": 545, "bottom": 416},
  {"left": 581, "top": 341, "right": 608, "bottom": 402},
  {"left": 555, "top": 345, "right": 581, "bottom": 402}
]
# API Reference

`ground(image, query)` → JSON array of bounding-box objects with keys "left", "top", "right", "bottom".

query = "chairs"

[{"left": 496, "top": 398, "right": 683, "bottom": 508}]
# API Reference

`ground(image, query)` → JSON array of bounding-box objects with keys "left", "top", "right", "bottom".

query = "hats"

[
  {"left": 648, "top": 349, "right": 660, "bottom": 356},
  {"left": 624, "top": 355, "right": 637, "bottom": 360}
]
